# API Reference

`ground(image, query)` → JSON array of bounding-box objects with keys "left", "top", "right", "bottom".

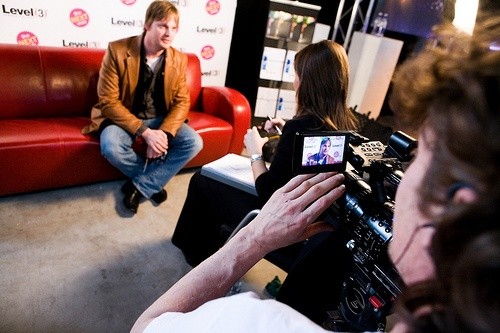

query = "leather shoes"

[
  {"left": 151, "top": 189, "right": 166, "bottom": 205},
  {"left": 121, "top": 180, "right": 140, "bottom": 213}
]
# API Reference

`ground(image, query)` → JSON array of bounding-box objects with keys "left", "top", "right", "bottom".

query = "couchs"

[{"left": 0, "top": 43, "right": 251, "bottom": 196}]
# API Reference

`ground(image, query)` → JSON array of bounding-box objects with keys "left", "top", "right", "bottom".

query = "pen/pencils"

[{"left": 267, "top": 115, "right": 282, "bottom": 135}]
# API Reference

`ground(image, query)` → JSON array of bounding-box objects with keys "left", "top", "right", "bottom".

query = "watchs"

[{"left": 250, "top": 153, "right": 265, "bottom": 166}]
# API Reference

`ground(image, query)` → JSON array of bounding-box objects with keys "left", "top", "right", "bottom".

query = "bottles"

[{"left": 370, "top": 11, "right": 389, "bottom": 37}]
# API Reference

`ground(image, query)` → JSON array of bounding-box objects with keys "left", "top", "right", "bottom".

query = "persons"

[
  {"left": 305, "top": 138, "right": 335, "bottom": 165},
  {"left": 126, "top": 10, "right": 500, "bottom": 331},
  {"left": 82, "top": 0, "right": 204, "bottom": 213},
  {"left": 242, "top": 39, "right": 361, "bottom": 202}
]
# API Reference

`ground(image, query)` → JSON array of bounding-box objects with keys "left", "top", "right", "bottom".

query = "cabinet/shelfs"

[{"left": 223, "top": 0, "right": 322, "bottom": 133}]
